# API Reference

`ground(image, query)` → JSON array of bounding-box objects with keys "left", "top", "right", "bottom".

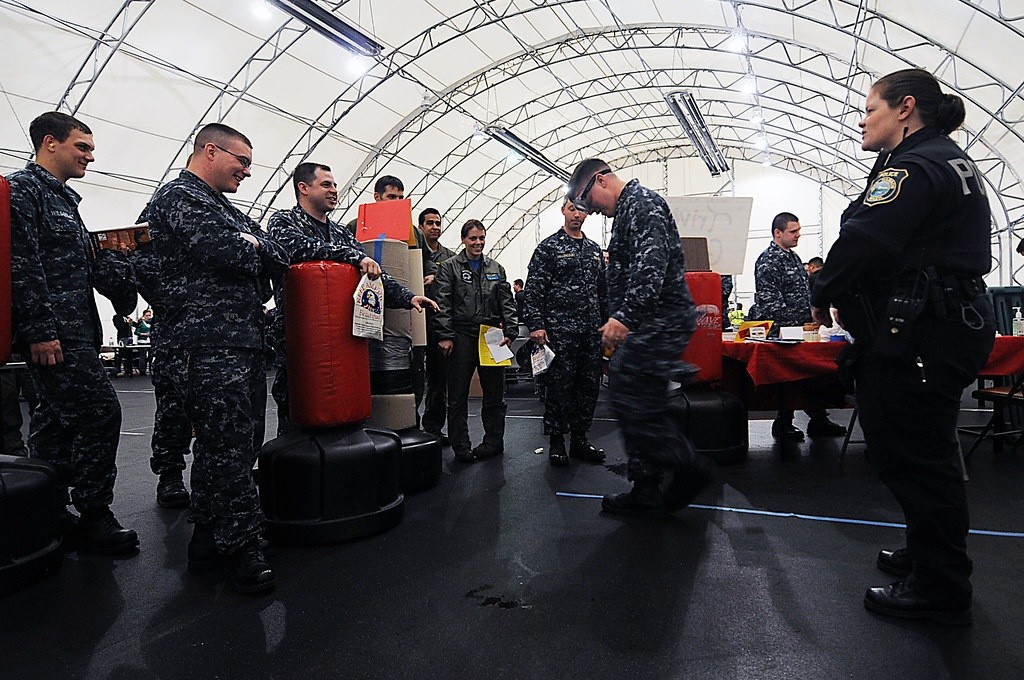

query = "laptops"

[{"left": 679, "top": 238, "right": 713, "bottom": 273}]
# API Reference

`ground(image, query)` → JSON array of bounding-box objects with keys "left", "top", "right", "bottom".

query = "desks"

[
  {"left": 98, "top": 343, "right": 152, "bottom": 376},
  {"left": 721, "top": 335, "right": 1024, "bottom": 452}
]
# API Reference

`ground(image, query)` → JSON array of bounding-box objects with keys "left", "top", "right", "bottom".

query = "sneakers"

[
  {"left": 474, "top": 441, "right": 500, "bottom": 458},
  {"left": 79, "top": 509, "right": 141, "bottom": 550},
  {"left": 186, "top": 538, "right": 237, "bottom": 569},
  {"left": 450, "top": 448, "right": 472, "bottom": 471},
  {"left": 235, "top": 545, "right": 276, "bottom": 595},
  {"left": 155, "top": 476, "right": 192, "bottom": 509},
  {"left": 549, "top": 444, "right": 570, "bottom": 466},
  {"left": 862, "top": 573, "right": 969, "bottom": 626},
  {"left": 570, "top": 440, "right": 607, "bottom": 461},
  {"left": 879, "top": 546, "right": 911, "bottom": 575}
]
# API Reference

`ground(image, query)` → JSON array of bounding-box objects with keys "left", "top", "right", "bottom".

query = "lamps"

[
  {"left": 483, "top": 125, "right": 573, "bottom": 187},
  {"left": 669, "top": 91, "right": 732, "bottom": 175},
  {"left": 275, "top": 0, "right": 385, "bottom": 58}
]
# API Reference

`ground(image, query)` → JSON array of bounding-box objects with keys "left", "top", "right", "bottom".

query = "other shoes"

[
  {"left": 650, "top": 458, "right": 716, "bottom": 517},
  {"left": 771, "top": 418, "right": 805, "bottom": 441},
  {"left": 602, "top": 488, "right": 652, "bottom": 518},
  {"left": 425, "top": 427, "right": 451, "bottom": 446},
  {"left": 805, "top": 417, "right": 848, "bottom": 437}
]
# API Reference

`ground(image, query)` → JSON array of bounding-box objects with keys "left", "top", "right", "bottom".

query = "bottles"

[{"left": 109, "top": 337, "right": 114, "bottom": 346}]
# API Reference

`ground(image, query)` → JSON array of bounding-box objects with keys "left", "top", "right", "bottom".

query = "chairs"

[
  {"left": 961, "top": 368, "right": 1023, "bottom": 466},
  {"left": 838, "top": 379, "right": 970, "bottom": 482}
]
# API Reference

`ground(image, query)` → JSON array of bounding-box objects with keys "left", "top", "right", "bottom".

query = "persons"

[
  {"left": 720, "top": 275, "right": 748, "bottom": 332},
  {"left": 136, "top": 123, "right": 290, "bottom": 600},
  {"left": 523, "top": 194, "right": 608, "bottom": 462},
  {"left": 346, "top": 175, "right": 436, "bottom": 286},
  {"left": 134, "top": 310, "right": 152, "bottom": 375},
  {"left": 112, "top": 314, "right": 138, "bottom": 375},
  {"left": 432, "top": 219, "right": 519, "bottom": 461},
  {"left": 514, "top": 279, "right": 524, "bottom": 322},
  {"left": 0, "top": 112, "right": 141, "bottom": 551},
  {"left": 809, "top": 69, "right": 996, "bottom": 629},
  {"left": 568, "top": 159, "right": 696, "bottom": 516},
  {"left": 749, "top": 212, "right": 846, "bottom": 440},
  {"left": 418, "top": 208, "right": 457, "bottom": 446},
  {"left": 602, "top": 250, "right": 609, "bottom": 265},
  {"left": 1016, "top": 238, "right": 1024, "bottom": 256},
  {"left": 268, "top": 163, "right": 440, "bottom": 438}
]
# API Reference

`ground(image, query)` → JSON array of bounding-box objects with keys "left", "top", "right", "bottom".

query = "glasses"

[
  {"left": 574, "top": 199, "right": 590, "bottom": 213},
  {"left": 202, "top": 145, "right": 250, "bottom": 171}
]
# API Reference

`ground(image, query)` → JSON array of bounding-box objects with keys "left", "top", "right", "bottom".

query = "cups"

[{"left": 802, "top": 331, "right": 818, "bottom": 342}]
